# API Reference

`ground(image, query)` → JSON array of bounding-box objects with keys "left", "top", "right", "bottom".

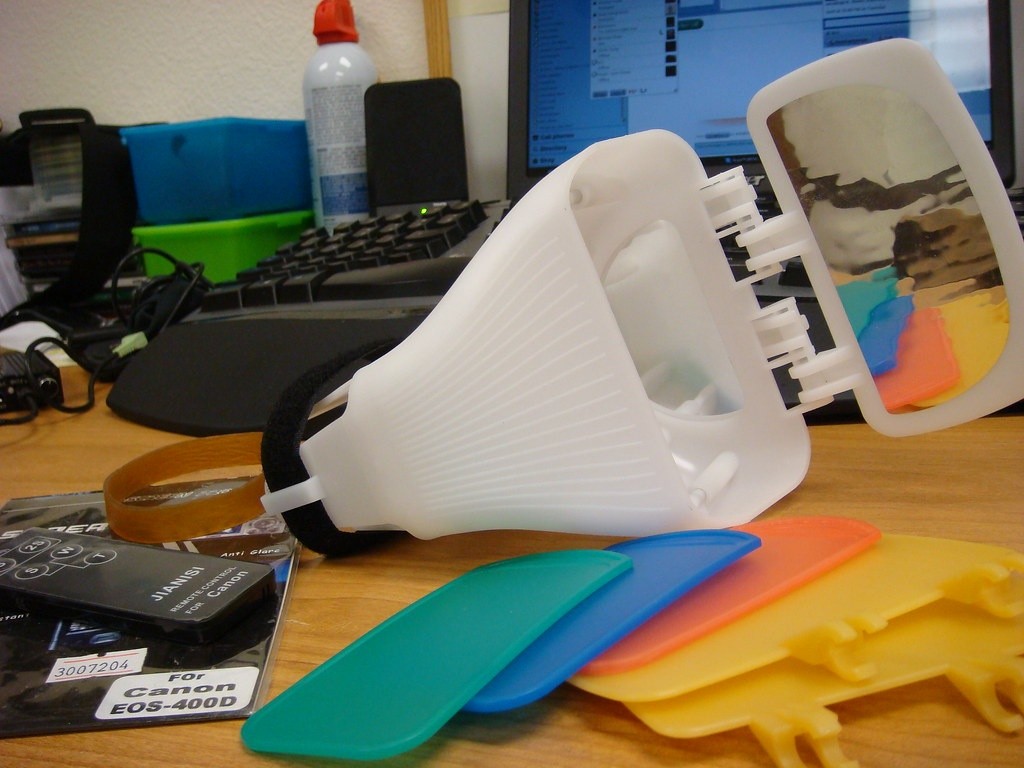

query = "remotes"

[{"left": 0, "top": 526, "right": 275, "bottom": 643}]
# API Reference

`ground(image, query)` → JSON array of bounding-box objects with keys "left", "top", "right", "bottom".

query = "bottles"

[{"left": 304, "top": 0, "right": 380, "bottom": 237}]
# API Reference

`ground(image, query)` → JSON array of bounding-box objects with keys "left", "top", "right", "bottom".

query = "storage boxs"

[
  {"left": 121, "top": 116, "right": 313, "bottom": 225},
  {"left": 132, "top": 212, "right": 312, "bottom": 283}
]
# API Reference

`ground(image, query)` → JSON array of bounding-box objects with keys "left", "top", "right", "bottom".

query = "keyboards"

[{"left": 106, "top": 191, "right": 1023, "bottom": 437}]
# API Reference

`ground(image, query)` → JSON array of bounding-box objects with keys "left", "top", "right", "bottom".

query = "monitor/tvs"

[{"left": 506, "top": 1, "right": 1015, "bottom": 208}]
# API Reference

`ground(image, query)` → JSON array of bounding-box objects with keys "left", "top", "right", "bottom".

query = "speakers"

[{"left": 363, "top": 76, "right": 469, "bottom": 219}]
